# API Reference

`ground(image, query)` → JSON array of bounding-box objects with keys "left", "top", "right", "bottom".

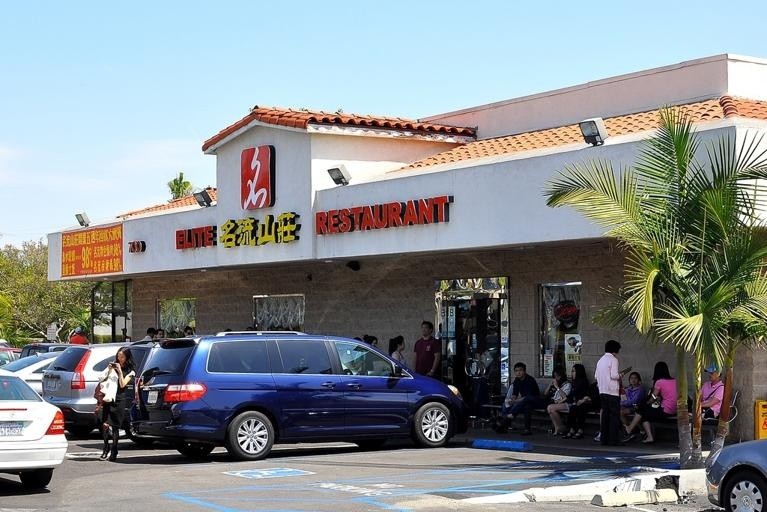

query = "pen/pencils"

[{"left": 128, "top": 329, "right": 468, "bottom": 459}]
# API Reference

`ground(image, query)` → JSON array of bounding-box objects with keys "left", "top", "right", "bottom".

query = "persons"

[
  {"left": 489, "top": 340, "right": 678, "bottom": 446},
  {"left": 68, "top": 327, "right": 89, "bottom": 345},
  {"left": 100, "top": 347, "right": 135, "bottom": 463},
  {"left": 688, "top": 365, "right": 724, "bottom": 450},
  {"left": 140, "top": 325, "right": 300, "bottom": 341},
  {"left": 342, "top": 321, "right": 441, "bottom": 382}
]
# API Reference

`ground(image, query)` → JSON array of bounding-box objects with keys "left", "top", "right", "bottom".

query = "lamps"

[{"left": 479, "top": 381, "right": 741, "bottom": 447}]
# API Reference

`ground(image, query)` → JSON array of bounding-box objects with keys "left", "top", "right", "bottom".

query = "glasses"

[
  {"left": 576, "top": 116, "right": 609, "bottom": 146},
  {"left": 326, "top": 162, "right": 351, "bottom": 188},
  {"left": 192, "top": 189, "right": 214, "bottom": 209},
  {"left": 74, "top": 211, "right": 90, "bottom": 229}
]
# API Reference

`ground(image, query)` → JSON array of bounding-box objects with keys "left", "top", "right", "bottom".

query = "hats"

[
  {"left": 0, "top": 340, "right": 139, "bottom": 492},
  {"left": 703, "top": 437, "right": 767, "bottom": 512}
]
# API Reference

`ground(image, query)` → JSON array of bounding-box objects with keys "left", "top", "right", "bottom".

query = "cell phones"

[{"left": 110, "top": 362, "right": 118, "bottom": 369}]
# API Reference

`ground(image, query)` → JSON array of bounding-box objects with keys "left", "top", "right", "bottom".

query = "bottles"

[{"left": 704, "top": 362, "right": 717, "bottom": 374}]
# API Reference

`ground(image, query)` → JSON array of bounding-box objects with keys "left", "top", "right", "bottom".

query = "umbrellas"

[{"left": 100, "top": 447, "right": 118, "bottom": 460}]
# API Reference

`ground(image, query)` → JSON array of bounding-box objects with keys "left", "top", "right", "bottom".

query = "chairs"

[{"left": 490, "top": 418, "right": 656, "bottom": 443}]
